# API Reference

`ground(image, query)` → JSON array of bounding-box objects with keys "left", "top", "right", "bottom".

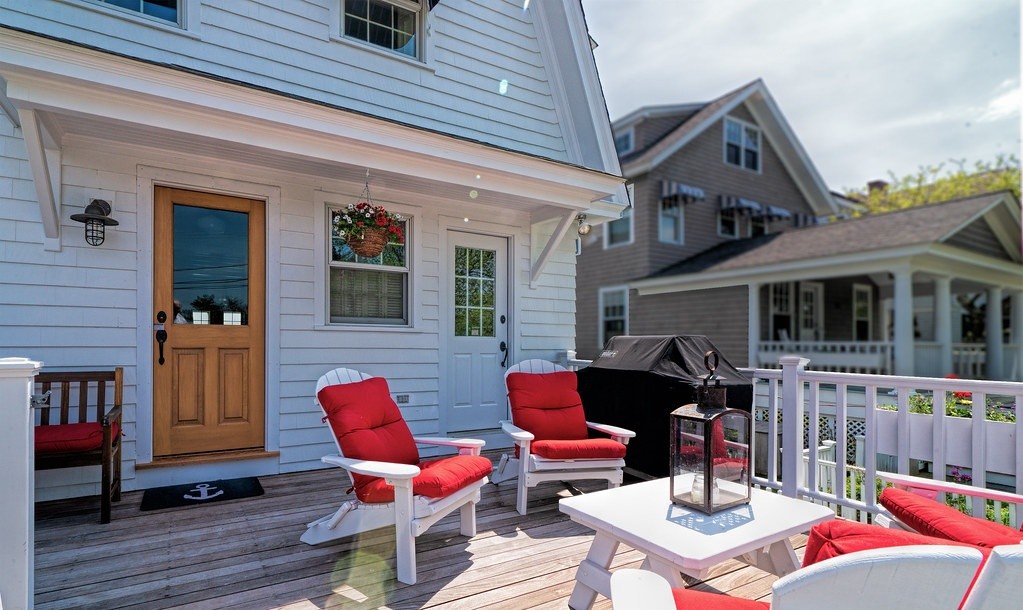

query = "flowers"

[
  {"left": 334, "top": 203, "right": 406, "bottom": 244},
  {"left": 876, "top": 373, "right": 1017, "bottom": 423}
]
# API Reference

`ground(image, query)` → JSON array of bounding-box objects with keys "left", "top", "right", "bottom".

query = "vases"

[
  {"left": 877, "top": 408, "right": 1017, "bottom": 477},
  {"left": 346, "top": 229, "right": 391, "bottom": 257}
]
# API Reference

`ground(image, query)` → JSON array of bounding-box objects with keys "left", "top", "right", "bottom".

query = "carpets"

[{"left": 140, "top": 476, "right": 265, "bottom": 511}]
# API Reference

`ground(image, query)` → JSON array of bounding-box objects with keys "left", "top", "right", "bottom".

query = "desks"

[{"left": 559, "top": 472, "right": 835, "bottom": 610}]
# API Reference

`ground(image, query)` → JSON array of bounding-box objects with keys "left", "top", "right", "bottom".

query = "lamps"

[
  {"left": 669, "top": 351, "right": 751, "bottom": 514},
  {"left": 577, "top": 214, "right": 591, "bottom": 236},
  {"left": 70, "top": 188, "right": 119, "bottom": 247}
]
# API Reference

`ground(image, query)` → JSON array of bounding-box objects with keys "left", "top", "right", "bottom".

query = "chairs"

[
  {"left": 35, "top": 367, "right": 126, "bottom": 524},
  {"left": 491, "top": 359, "right": 636, "bottom": 516},
  {"left": 611, "top": 486, "right": 1022, "bottom": 610},
  {"left": 299, "top": 368, "right": 493, "bottom": 585}
]
